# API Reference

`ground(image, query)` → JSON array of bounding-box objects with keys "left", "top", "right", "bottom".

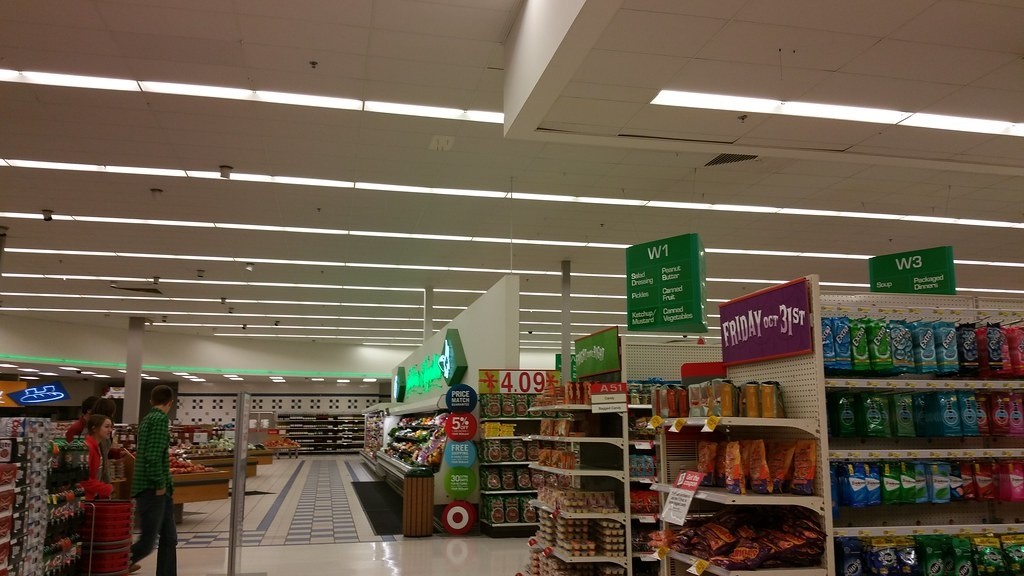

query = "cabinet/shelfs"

[
  {"left": 648, "top": 274, "right": 1024, "bottom": 576},
  {"left": 479, "top": 417, "right": 539, "bottom": 538},
  {"left": 275, "top": 418, "right": 365, "bottom": 455},
  {"left": 525, "top": 333, "right": 723, "bottom": 576}
]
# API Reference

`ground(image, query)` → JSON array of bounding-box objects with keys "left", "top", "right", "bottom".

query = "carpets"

[
  {"left": 351, "top": 480, "right": 442, "bottom": 536},
  {"left": 228, "top": 489, "right": 277, "bottom": 497}
]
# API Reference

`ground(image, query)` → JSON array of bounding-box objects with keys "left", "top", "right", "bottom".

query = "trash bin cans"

[{"left": 403, "top": 467, "right": 435, "bottom": 537}]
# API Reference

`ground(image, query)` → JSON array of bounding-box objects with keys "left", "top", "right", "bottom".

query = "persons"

[
  {"left": 131, "top": 385, "right": 185, "bottom": 576},
  {"left": 66, "top": 395, "right": 142, "bottom": 573}
]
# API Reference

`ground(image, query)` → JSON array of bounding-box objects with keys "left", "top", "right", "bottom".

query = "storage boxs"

[
  {"left": 530, "top": 380, "right": 600, "bottom": 470},
  {"left": 0, "top": 417, "right": 51, "bottom": 576}
]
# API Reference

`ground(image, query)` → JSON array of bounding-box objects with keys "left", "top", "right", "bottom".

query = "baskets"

[{"left": 77, "top": 499, "right": 136, "bottom": 576}]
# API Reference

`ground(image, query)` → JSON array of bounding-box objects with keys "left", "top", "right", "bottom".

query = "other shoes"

[{"left": 129, "top": 565, "right": 141, "bottom": 572}]
